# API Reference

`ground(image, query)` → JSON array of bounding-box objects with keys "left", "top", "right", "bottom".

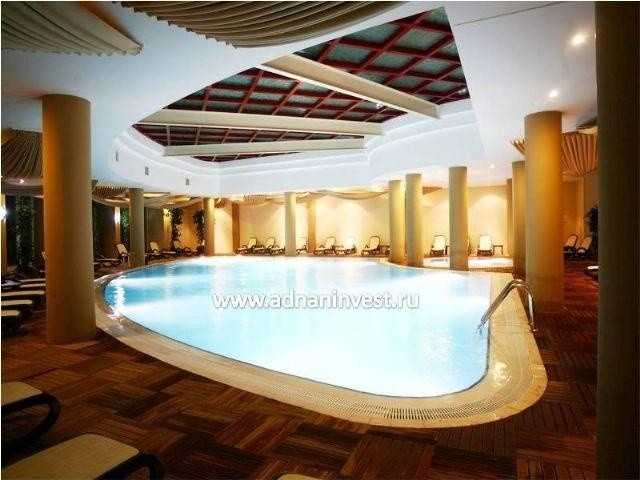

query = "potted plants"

[
  {"left": 192, "top": 210, "right": 206, "bottom": 254},
  {"left": 584, "top": 205, "right": 599, "bottom": 261},
  {"left": 10, "top": 192, "right": 35, "bottom": 276},
  {"left": 169, "top": 209, "right": 183, "bottom": 251}
]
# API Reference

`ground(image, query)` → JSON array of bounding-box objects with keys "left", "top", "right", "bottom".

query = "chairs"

[
  {"left": 235, "top": 236, "right": 380, "bottom": 256},
  {"left": 430, "top": 235, "right": 493, "bottom": 258},
  {"left": 1, "top": 269, "right": 47, "bottom": 334},
  {"left": 562, "top": 233, "right": 594, "bottom": 260},
  {"left": 37, "top": 240, "right": 192, "bottom": 276}
]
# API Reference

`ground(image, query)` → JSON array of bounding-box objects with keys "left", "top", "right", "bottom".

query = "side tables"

[
  {"left": 493, "top": 244, "right": 504, "bottom": 255},
  {"left": 192, "top": 249, "right": 198, "bottom": 255},
  {"left": 379, "top": 244, "right": 390, "bottom": 255}
]
